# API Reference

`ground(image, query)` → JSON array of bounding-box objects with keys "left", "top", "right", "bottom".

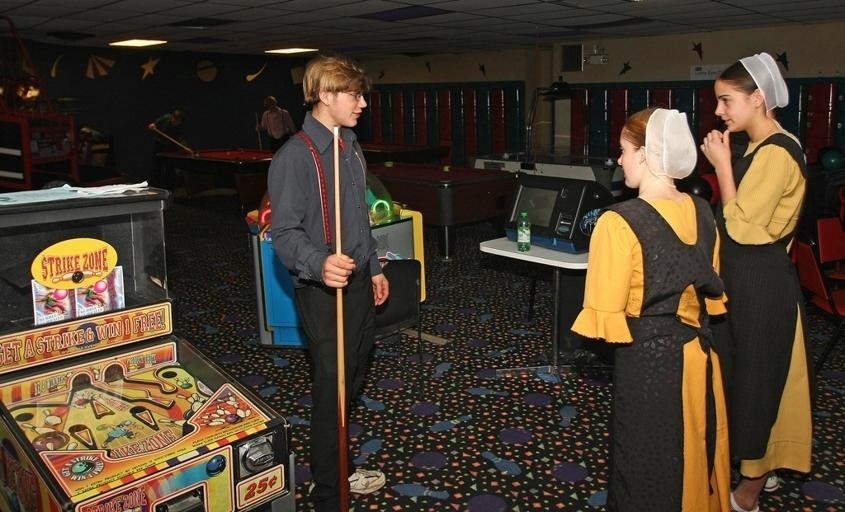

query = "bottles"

[{"left": 517, "top": 213, "right": 532, "bottom": 252}]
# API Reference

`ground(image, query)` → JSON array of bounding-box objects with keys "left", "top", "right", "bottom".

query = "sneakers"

[
  {"left": 307, "top": 467, "right": 387, "bottom": 496},
  {"left": 730, "top": 490, "right": 761, "bottom": 512},
  {"left": 763, "top": 474, "right": 781, "bottom": 493}
]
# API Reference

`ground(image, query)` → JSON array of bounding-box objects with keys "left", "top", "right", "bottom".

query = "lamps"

[{"left": 522, "top": 81, "right": 572, "bottom": 159}]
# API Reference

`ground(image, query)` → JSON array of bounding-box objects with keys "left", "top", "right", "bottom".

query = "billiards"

[
  {"left": 252, "top": 156, "right": 257, "bottom": 160},
  {"left": 226, "top": 152, "right": 231, "bottom": 156},
  {"left": 196, "top": 154, "right": 200, "bottom": 157}
]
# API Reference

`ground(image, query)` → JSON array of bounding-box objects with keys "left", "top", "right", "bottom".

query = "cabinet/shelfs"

[{"left": 0, "top": 106, "right": 83, "bottom": 192}]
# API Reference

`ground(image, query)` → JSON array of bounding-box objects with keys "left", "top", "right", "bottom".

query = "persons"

[
  {"left": 255, "top": 96, "right": 298, "bottom": 153},
  {"left": 268, "top": 52, "right": 392, "bottom": 512},
  {"left": 570, "top": 105, "right": 731, "bottom": 512},
  {"left": 147, "top": 108, "right": 184, "bottom": 191},
  {"left": 701, "top": 52, "right": 815, "bottom": 512}
]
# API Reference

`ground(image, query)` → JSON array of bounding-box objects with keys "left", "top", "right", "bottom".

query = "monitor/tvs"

[
  {"left": 561, "top": 43, "right": 584, "bottom": 72},
  {"left": 506, "top": 174, "right": 616, "bottom": 254}
]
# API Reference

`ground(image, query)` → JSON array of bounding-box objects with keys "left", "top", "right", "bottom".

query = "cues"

[
  {"left": 151, "top": 124, "right": 197, "bottom": 156},
  {"left": 255, "top": 110, "right": 263, "bottom": 151},
  {"left": 330, "top": 125, "right": 349, "bottom": 511}
]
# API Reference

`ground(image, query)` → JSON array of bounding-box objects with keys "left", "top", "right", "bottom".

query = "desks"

[
  {"left": 155, "top": 149, "right": 275, "bottom": 205},
  {"left": 367, "top": 161, "right": 517, "bottom": 265},
  {"left": 478, "top": 235, "right": 615, "bottom": 386},
  {"left": 359, "top": 143, "right": 450, "bottom": 162}
]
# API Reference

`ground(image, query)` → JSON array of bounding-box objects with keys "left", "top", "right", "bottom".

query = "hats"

[
  {"left": 644, "top": 107, "right": 699, "bottom": 181},
  {"left": 737, "top": 51, "right": 792, "bottom": 114}
]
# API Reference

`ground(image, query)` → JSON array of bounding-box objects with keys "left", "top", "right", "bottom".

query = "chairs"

[
  {"left": 372, "top": 258, "right": 429, "bottom": 371},
  {"left": 792, "top": 212, "right": 844, "bottom": 378}
]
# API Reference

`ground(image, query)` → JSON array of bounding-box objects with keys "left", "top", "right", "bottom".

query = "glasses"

[{"left": 337, "top": 89, "right": 362, "bottom": 101}]
